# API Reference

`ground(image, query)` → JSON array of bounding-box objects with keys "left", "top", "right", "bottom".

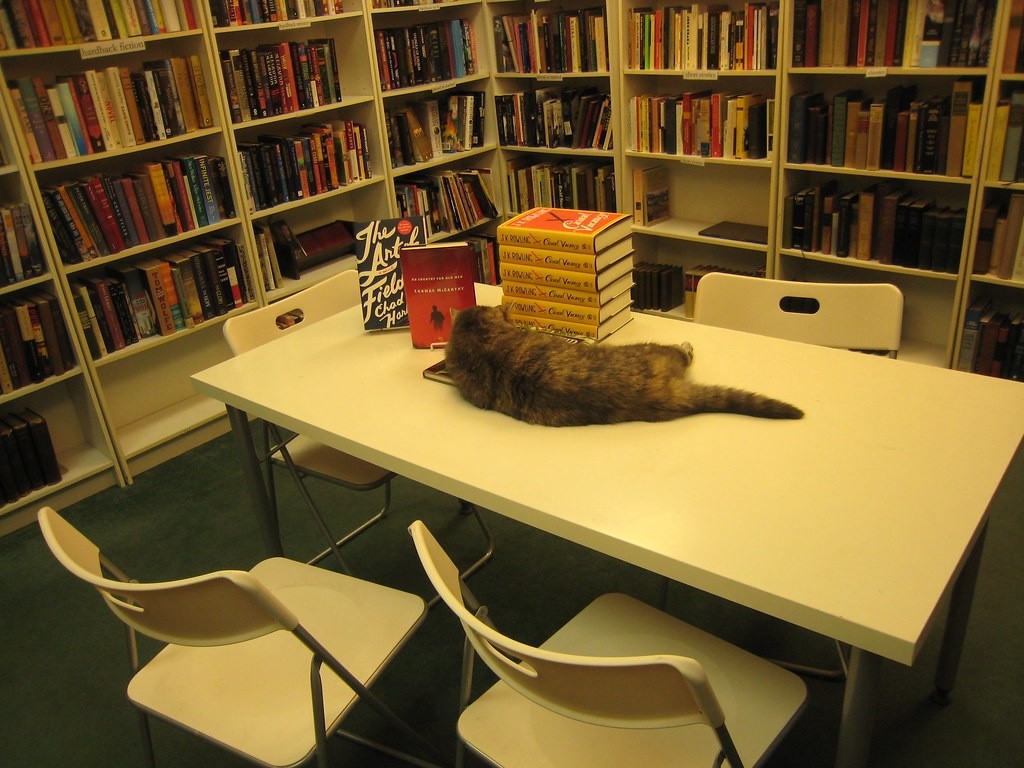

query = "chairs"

[
  {"left": 695, "top": 273, "right": 903, "bottom": 359},
  {"left": 409, "top": 519, "right": 807, "bottom": 768},
  {"left": 223, "top": 270, "right": 492, "bottom": 614},
  {"left": 40, "top": 505, "right": 428, "bottom": 768}
]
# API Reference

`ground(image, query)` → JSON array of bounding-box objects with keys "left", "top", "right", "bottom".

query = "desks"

[{"left": 191, "top": 283, "right": 1024, "bottom": 768}]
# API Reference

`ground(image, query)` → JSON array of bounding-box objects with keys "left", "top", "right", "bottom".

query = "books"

[
  {"left": 371, "top": 0, "right": 502, "bottom": 285},
  {"left": 208, "top": 0, "right": 372, "bottom": 291},
  {"left": 492, "top": 9, "right": 617, "bottom": 213},
  {"left": 423, "top": 323, "right": 597, "bottom": 386},
  {"left": 0, "top": 0, "right": 256, "bottom": 507},
  {"left": 399, "top": 242, "right": 478, "bottom": 348},
  {"left": 957, "top": 0, "right": 1024, "bottom": 380},
  {"left": 497, "top": 207, "right": 635, "bottom": 343},
  {"left": 632, "top": 3, "right": 779, "bottom": 319},
  {"left": 783, "top": 1, "right": 998, "bottom": 356},
  {"left": 354, "top": 216, "right": 427, "bottom": 330}
]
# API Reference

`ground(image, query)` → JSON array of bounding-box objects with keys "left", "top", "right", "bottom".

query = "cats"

[{"left": 441, "top": 300, "right": 806, "bottom": 429}]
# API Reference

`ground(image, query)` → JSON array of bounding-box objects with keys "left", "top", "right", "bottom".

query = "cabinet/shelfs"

[
  {"left": 482, "top": 0, "right": 622, "bottom": 215},
  {"left": 365, "top": 0, "right": 506, "bottom": 256},
  {"left": 0, "top": 0, "right": 263, "bottom": 482},
  {"left": 774, "top": 12, "right": 1004, "bottom": 369},
  {"left": 952, "top": 0, "right": 1024, "bottom": 381},
  {"left": 622, "top": 1, "right": 785, "bottom": 277},
  {"left": 0, "top": 87, "right": 131, "bottom": 535},
  {"left": 203, "top": 0, "right": 394, "bottom": 306}
]
